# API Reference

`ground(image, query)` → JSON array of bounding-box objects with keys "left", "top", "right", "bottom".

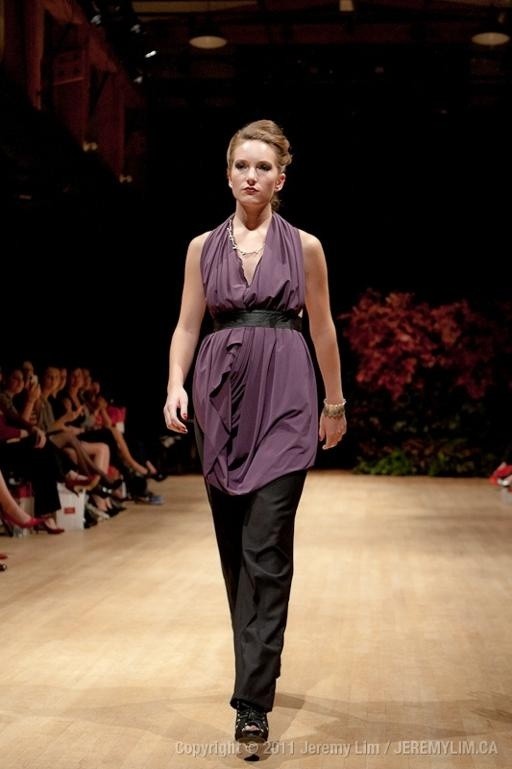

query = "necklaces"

[{"left": 226, "top": 212, "right": 265, "bottom": 256}]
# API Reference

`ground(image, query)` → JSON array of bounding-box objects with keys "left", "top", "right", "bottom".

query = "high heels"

[
  {"left": 0, "top": 467, "right": 168, "bottom": 571},
  {"left": 235, "top": 707, "right": 270, "bottom": 744}
]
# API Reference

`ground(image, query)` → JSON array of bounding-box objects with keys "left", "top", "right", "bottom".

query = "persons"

[
  {"left": 162, "top": 118, "right": 349, "bottom": 745},
  {"left": 0, "top": 353, "right": 169, "bottom": 572}
]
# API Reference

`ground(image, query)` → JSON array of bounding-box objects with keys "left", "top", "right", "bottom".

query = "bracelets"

[{"left": 322, "top": 398, "right": 347, "bottom": 418}]
